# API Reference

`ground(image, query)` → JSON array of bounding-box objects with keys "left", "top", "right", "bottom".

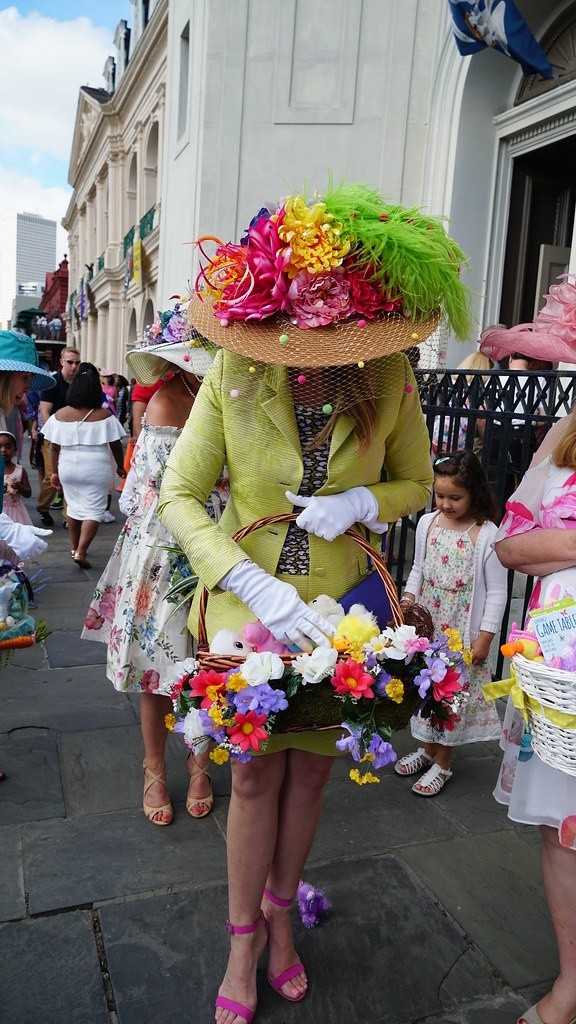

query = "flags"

[{"left": 447, "top": 0, "right": 553, "bottom": 79}]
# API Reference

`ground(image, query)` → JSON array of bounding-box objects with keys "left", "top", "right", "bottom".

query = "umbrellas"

[{"left": 13, "top": 307, "right": 47, "bottom": 333}]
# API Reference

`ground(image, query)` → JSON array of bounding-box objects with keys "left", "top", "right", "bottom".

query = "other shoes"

[
  {"left": 37, "top": 483, "right": 115, "bottom": 568},
  {"left": 517, "top": 993, "right": 576, "bottom": 1024}
]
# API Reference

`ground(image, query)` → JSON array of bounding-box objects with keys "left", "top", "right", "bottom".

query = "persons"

[
  {"left": 132, "top": 379, "right": 165, "bottom": 438},
  {"left": 0, "top": 327, "right": 57, "bottom": 568},
  {"left": 37, "top": 348, "right": 80, "bottom": 528},
  {"left": 99, "top": 369, "right": 136, "bottom": 492},
  {"left": 394, "top": 451, "right": 507, "bottom": 796},
  {"left": 79, "top": 299, "right": 230, "bottom": 825},
  {"left": 431, "top": 353, "right": 492, "bottom": 459},
  {"left": 41, "top": 364, "right": 126, "bottom": 568},
  {"left": 31, "top": 316, "right": 62, "bottom": 340},
  {"left": 158, "top": 188, "right": 467, "bottom": 1024},
  {"left": 482, "top": 353, "right": 548, "bottom": 501},
  {"left": 485, "top": 273, "right": 576, "bottom": 1024}
]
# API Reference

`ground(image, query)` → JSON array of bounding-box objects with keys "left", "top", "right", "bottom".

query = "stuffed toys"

[
  {"left": 500, "top": 640, "right": 543, "bottom": 662},
  {"left": 210, "top": 593, "right": 377, "bottom": 657}
]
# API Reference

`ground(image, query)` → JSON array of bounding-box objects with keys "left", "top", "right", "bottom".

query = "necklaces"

[{"left": 180, "top": 375, "right": 195, "bottom": 398}]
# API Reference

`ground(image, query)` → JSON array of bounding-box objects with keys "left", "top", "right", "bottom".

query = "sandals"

[
  {"left": 410, "top": 760, "right": 453, "bottom": 796},
  {"left": 392, "top": 746, "right": 439, "bottom": 776}
]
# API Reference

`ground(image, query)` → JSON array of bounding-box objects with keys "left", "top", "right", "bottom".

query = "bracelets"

[{"left": 400, "top": 596, "right": 412, "bottom": 603}]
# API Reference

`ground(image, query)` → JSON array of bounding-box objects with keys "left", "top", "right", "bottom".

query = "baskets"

[
  {"left": 0, "top": 559, "right": 35, "bottom": 673},
  {"left": 168, "top": 509, "right": 420, "bottom": 736},
  {"left": 397, "top": 600, "right": 435, "bottom": 641},
  {"left": 510, "top": 652, "right": 576, "bottom": 777}
]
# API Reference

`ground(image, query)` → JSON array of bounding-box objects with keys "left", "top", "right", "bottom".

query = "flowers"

[
  {"left": 142, "top": 295, "right": 190, "bottom": 342},
  {"left": 195, "top": 196, "right": 403, "bottom": 329},
  {"left": 148, "top": 624, "right": 472, "bottom": 785}
]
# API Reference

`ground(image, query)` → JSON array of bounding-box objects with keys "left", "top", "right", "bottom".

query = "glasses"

[{"left": 62, "top": 359, "right": 81, "bottom": 365}]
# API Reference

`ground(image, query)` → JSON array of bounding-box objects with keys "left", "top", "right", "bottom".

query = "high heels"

[
  {"left": 261, "top": 888, "right": 307, "bottom": 1003},
  {"left": 214, "top": 910, "right": 271, "bottom": 1024},
  {"left": 141, "top": 756, "right": 173, "bottom": 826},
  {"left": 185, "top": 752, "right": 214, "bottom": 818}
]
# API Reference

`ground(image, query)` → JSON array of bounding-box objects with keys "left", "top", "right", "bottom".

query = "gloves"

[
  {"left": 216, "top": 561, "right": 338, "bottom": 651},
  {"left": 0, "top": 512, "right": 53, "bottom": 560},
  {"left": 283, "top": 486, "right": 379, "bottom": 542}
]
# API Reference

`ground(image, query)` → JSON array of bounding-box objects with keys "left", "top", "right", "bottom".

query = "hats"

[
  {"left": 0, "top": 328, "right": 56, "bottom": 391},
  {"left": 99, "top": 369, "right": 118, "bottom": 383},
  {"left": 125, "top": 299, "right": 216, "bottom": 387},
  {"left": 186, "top": 186, "right": 475, "bottom": 369},
  {"left": 478, "top": 273, "right": 576, "bottom": 363}
]
